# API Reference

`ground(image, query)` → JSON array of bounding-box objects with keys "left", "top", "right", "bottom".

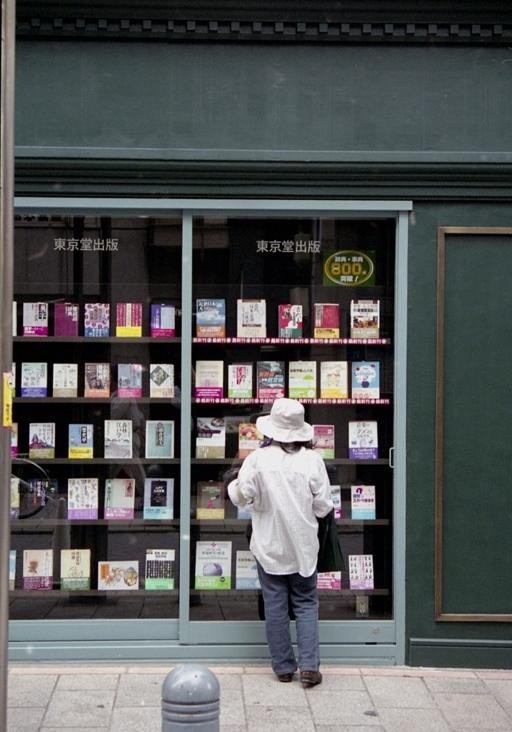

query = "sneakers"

[
  {"left": 278, "top": 670, "right": 295, "bottom": 686},
  {"left": 301, "top": 670, "right": 325, "bottom": 689}
]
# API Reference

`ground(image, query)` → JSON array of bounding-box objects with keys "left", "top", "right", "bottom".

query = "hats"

[{"left": 256, "top": 398, "right": 316, "bottom": 443}]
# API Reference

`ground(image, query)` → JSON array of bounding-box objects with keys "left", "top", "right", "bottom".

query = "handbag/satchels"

[{"left": 316, "top": 508, "right": 348, "bottom": 572}]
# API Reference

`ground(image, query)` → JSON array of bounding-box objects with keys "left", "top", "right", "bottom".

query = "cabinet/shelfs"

[
  {"left": 186, "top": 333, "right": 396, "bottom": 598},
  {"left": 7, "top": 329, "right": 184, "bottom": 602}
]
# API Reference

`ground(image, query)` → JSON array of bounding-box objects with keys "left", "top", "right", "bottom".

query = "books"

[
  {"left": 192, "top": 304, "right": 379, "bottom": 591},
  {"left": 13, "top": 298, "right": 179, "bottom": 590}
]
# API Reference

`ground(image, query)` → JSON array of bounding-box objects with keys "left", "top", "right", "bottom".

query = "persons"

[{"left": 222, "top": 397, "right": 335, "bottom": 687}]
